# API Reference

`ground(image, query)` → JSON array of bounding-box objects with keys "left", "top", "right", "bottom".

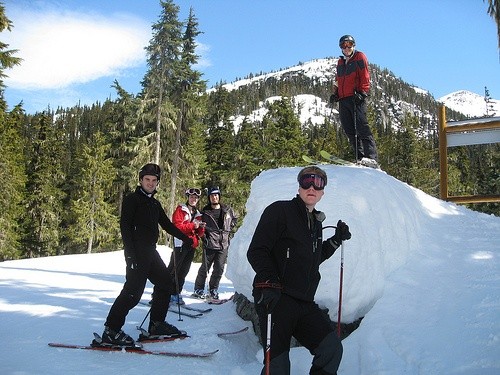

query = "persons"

[
  {"left": 247, "top": 166, "right": 352, "bottom": 375},
  {"left": 330, "top": 35, "right": 380, "bottom": 169},
  {"left": 166, "top": 187, "right": 237, "bottom": 306},
  {"left": 102, "top": 164, "right": 192, "bottom": 346}
]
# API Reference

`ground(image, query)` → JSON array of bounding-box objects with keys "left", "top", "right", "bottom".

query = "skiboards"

[
  {"left": 47, "top": 326, "right": 249, "bottom": 358},
  {"left": 138, "top": 301, "right": 213, "bottom": 317},
  {"left": 301, "top": 149, "right": 358, "bottom": 166},
  {"left": 192, "top": 295, "right": 234, "bottom": 305}
]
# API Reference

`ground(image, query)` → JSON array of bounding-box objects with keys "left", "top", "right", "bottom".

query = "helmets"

[
  {"left": 139, "top": 163, "right": 160, "bottom": 181},
  {"left": 207, "top": 188, "right": 221, "bottom": 203},
  {"left": 339, "top": 36, "right": 356, "bottom": 46}
]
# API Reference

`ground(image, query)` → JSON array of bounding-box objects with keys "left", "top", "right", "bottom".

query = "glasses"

[
  {"left": 144, "top": 164, "right": 160, "bottom": 174},
  {"left": 340, "top": 41, "right": 353, "bottom": 48},
  {"left": 188, "top": 188, "right": 201, "bottom": 195},
  {"left": 300, "top": 174, "right": 325, "bottom": 190}
]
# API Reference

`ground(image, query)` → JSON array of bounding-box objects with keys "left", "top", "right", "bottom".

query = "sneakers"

[
  {"left": 101, "top": 326, "right": 134, "bottom": 346},
  {"left": 171, "top": 294, "right": 184, "bottom": 305},
  {"left": 148, "top": 322, "right": 179, "bottom": 335}
]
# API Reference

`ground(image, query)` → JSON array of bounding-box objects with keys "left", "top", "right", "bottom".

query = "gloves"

[
  {"left": 329, "top": 95, "right": 338, "bottom": 103},
  {"left": 262, "top": 275, "right": 281, "bottom": 310},
  {"left": 180, "top": 234, "right": 193, "bottom": 246},
  {"left": 332, "top": 220, "right": 350, "bottom": 245},
  {"left": 353, "top": 91, "right": 366, "bottom": 103},
  {"left": 126, "top": 257, "right": 143, "bottom": 271}
]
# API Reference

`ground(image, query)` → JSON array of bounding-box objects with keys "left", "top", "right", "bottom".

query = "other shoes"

[
  {"left": 194, "top": 289, "right": 205, "bottom": 298},
  {"left": 360, "top": 158, "right": 377, "bottom": 168},
  {"left": 210, "top": 289, "right": 218, "bottom": 299}
]
